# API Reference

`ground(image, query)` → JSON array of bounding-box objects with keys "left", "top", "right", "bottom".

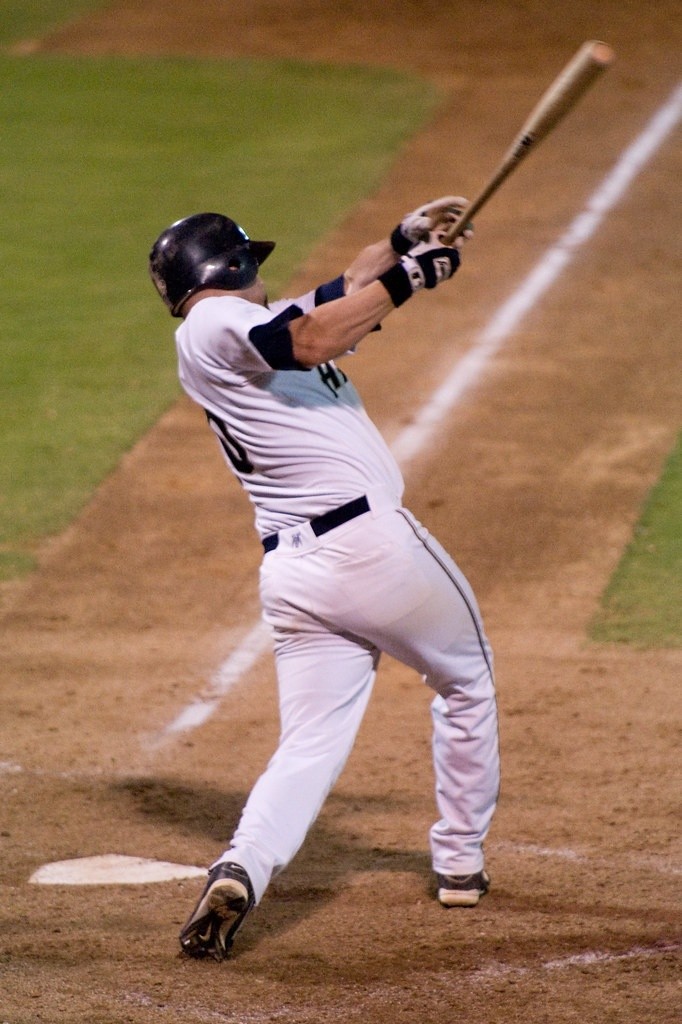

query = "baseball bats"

[{"left": 441, "top": 40, "right": 615, "bottom": 246}]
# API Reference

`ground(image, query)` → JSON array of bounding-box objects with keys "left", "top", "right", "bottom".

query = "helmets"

[{"left": 148, "top": 212, "right": 277, "bottom": 316}]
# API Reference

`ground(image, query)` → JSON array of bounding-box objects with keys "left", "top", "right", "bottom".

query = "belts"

[{"left": 262, "top": 495, "right": 371, "bottom": 552}]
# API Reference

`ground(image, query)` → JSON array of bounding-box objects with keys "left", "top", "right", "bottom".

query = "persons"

[{"left": 147, "top": 196, "right": 499, "bottom": 967}]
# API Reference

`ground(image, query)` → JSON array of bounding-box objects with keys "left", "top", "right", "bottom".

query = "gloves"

[
  {"left": 377, "top": 235, "right": 462, "bottom": 308},
  {"left": 390, "top": 194, "right": 474, "bottom": 256}
]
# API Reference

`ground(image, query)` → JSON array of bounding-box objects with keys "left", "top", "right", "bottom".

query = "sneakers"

[
  {"left": 179, "top": 863, "right": 256, "bottom": 960},
  {"left": 436, "top": 868, "right": 492, "bottom": 907}
]
